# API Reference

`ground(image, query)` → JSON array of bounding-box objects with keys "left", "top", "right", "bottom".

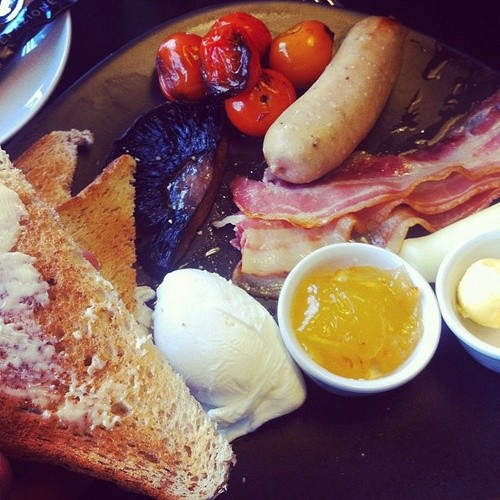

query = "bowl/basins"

[
  {"left": 277, "top": 243, "right": 441, "bottom": 396},
  {"left": 435, "top": 228, "right": 500, "bottom": 373}
]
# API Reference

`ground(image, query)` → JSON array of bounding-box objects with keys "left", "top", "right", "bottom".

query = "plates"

[
  {"left": 0, "top": 9, "right": 72, "bottom": 144},
  {"left": 4, "top": 1, "right": 500, "bottom": 500}
]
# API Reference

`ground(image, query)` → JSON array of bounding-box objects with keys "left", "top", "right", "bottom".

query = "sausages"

[{"left": 262, "top": 15, "right": 404, "bottom": 183}]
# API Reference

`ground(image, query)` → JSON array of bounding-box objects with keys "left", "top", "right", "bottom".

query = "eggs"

[{"left": 152, "top": 265, "right": 306, "bottom": 443}]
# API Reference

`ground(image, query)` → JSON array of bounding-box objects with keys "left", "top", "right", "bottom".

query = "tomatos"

[{"left": 154, "top": 12, "right": 334, "bottom": 137}]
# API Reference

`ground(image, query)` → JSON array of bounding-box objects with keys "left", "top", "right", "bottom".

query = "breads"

[{"left": 0, "top": 130, "right": 238, "bottom": 500}]
